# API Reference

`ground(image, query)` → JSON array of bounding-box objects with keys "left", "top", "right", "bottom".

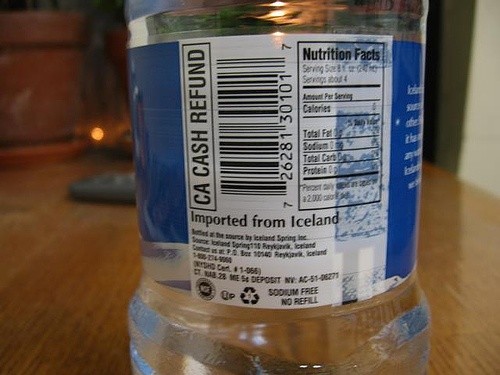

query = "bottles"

[{"left": 122, "top": 0, "right": 433, "bottom": 375}]
